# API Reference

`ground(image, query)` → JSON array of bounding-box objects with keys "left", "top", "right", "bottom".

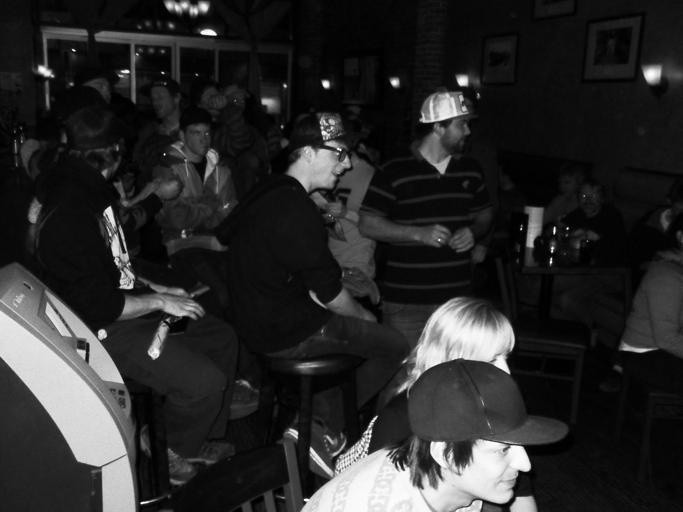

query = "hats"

[
  {"left": 65, "top": 105, "right": 137, "bottom": 152},
  {"left": 406, "top": 357, "right": 571, "bottom": 447},
  {"left": 418, "top": 89, "right": 480, "bottom": 125},
  {"left": 150, "top": 74, "right": 185, "bottom": 98},
  {"left": 268, "top": 108, "right": 348, "bottom": 166}
]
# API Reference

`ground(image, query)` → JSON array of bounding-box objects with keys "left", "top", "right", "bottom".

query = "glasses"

[{"left": 315, "top": 144, "right": 353, "bottom": 163}]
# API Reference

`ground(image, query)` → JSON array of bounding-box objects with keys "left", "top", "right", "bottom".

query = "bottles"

[
  {"left": 545, "top": 232, "right": 560, "bottom": 271},
  {"left": 11, "top": 124, "right": 25, "bottom": 156}
]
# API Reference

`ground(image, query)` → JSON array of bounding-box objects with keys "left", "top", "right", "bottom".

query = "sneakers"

[
  {"left": 283, "top": 427, "right": 335, "bottom": 480},
  {"left": 186, "top": 439, "right": 237, "bottom": 466},
  {"left": 229, "top": 378, "right": 278, "bottom": 410},
  {"left": 140, "top": 424, "right": 197, "bottom": 486},
  {"left": 322, "top": 430, "right": 348, "bottom": 461}
]
